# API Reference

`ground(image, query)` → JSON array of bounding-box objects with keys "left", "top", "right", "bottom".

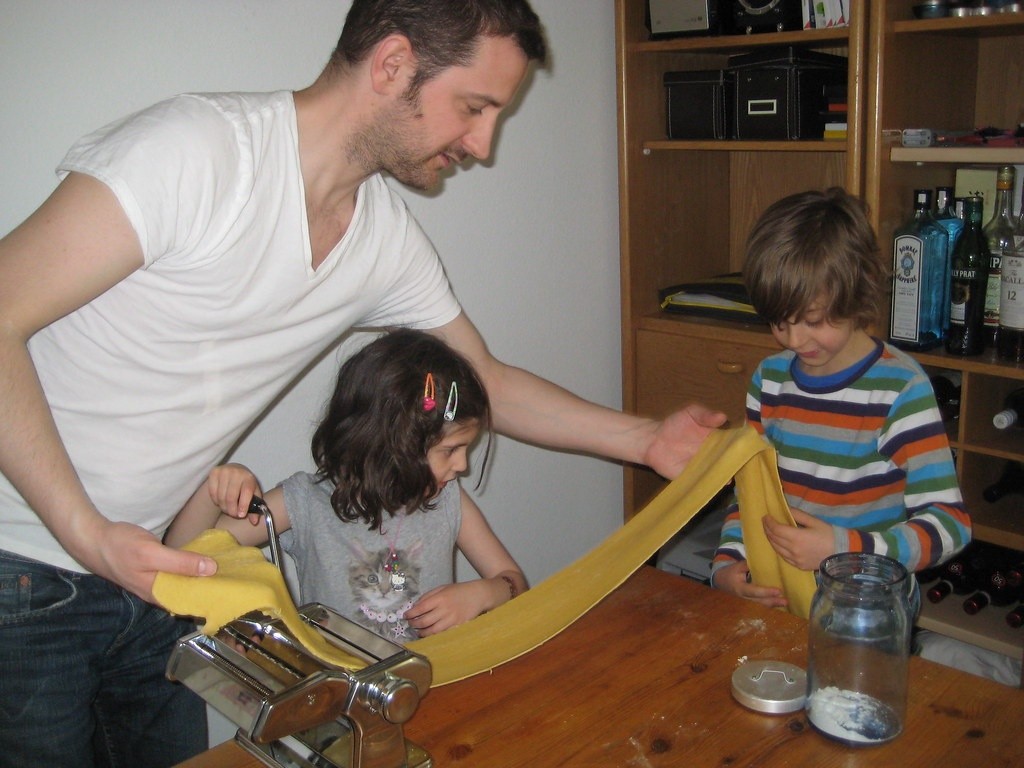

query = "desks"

[{"left": 177, "top": 563, "right": 1024, "bottom": 768}]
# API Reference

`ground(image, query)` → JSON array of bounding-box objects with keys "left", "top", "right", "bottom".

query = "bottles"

[
  {"left": 916, "top": 543, "right": 1024, "bottom": 631},
  {"left": 807, "top": 552, "right": 911, "bottom": 742},
  {"left": 992, "top": 387, "right": 1024, "bottom": 433},
  {"left": 983, "top": 462, "right": 1024, "bottom": 503},
  {"left": 889, "top": 165, "right": 1024, "bottom": 361},
  {"left": 927, "top": 368, "right": 962, "bottom": 420}
]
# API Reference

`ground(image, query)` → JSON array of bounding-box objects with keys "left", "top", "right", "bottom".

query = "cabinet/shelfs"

[{"left": 614, "top": 0, "right": 1024, "bottom": 690}]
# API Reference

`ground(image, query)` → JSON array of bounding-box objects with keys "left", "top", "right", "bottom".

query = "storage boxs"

[
  {"left": 662, "top": 70, "right": 737, "bottom": 141},
  {"left": 727, "top": 47, "right": 848, "bottom": 142}
]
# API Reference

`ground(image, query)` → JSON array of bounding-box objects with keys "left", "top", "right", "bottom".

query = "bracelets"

[{"left": 501, "top": 576, "right": 519, "bottom": 599}]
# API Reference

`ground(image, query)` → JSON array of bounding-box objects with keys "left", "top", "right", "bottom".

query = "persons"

[
  {"left": 707, "top": 190, "right": 973, "bottom": 653},
  {"left": 0, "top": 0, "right": 757, "bottom": 768},
  {"left": 162, "top": 327, "right": 531, "bottom": 645}
]
